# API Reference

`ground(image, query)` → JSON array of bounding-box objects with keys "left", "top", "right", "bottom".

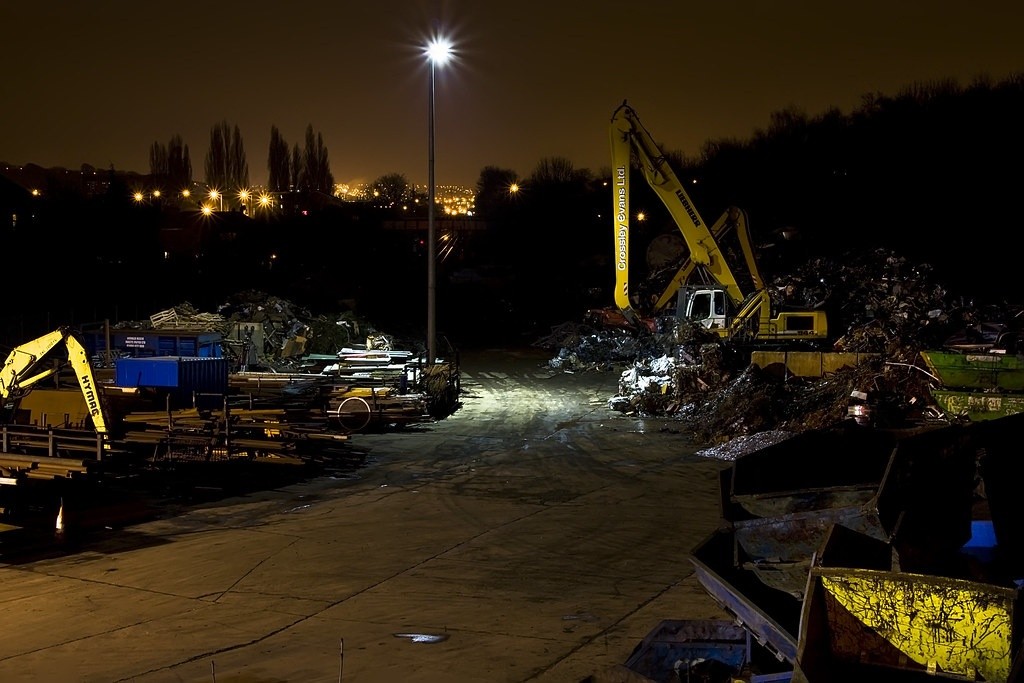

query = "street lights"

[{"left": 415, "top": 27, "right": 465, "bottom": 365}]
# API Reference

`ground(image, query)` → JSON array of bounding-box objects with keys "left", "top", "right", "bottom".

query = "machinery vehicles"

[
  {"left": 607, "top": 102, "right": 831, "bottom": 352},
  {"left": 1, "top": 325, "right": 138, "bottom": 538}
]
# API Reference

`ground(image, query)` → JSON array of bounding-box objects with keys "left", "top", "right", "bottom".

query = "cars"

[{"left": 586, "top": 306, "right": 654, "bottom": 337}]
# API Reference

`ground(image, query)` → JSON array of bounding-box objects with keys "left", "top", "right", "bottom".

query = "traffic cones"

[{"left": 45, "top": 495, "right": 75, "bottom": 550}]
